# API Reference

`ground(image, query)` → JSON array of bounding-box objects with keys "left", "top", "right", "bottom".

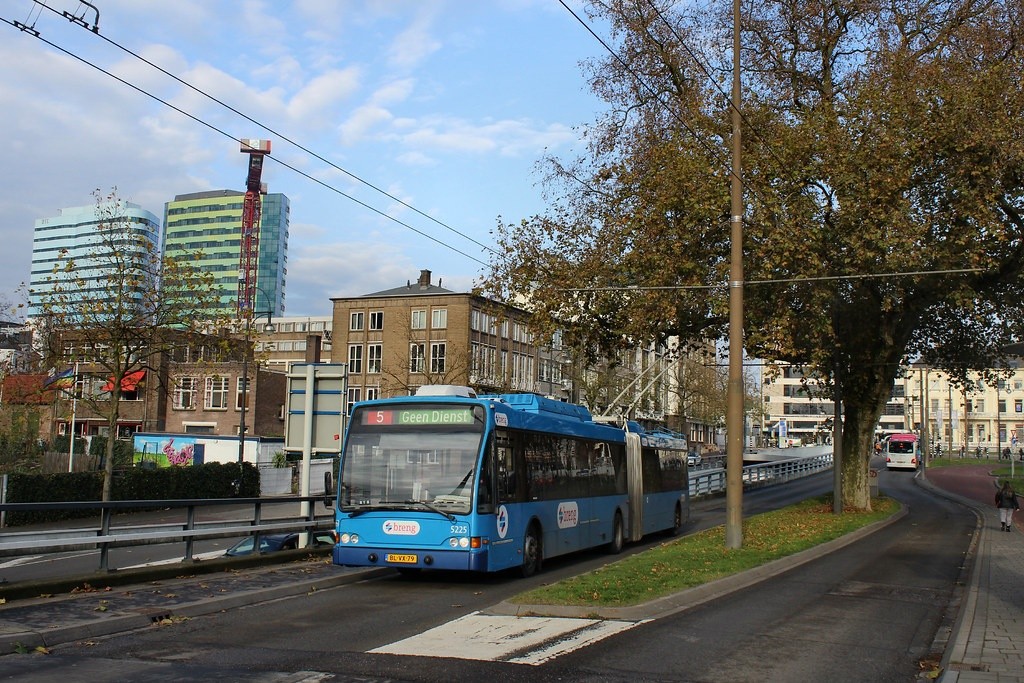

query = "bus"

[
  {"left": 882, "top": 412, "right": 921, "bottom": 470},
  {"left": 324, "top": 344, "right": 689, "bottom": 577}
]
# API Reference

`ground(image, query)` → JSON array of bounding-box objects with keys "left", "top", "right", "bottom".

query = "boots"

[
  {"left": 1001, "top": 522, "right": 1005, "bottom": 530},
  {"left": 1006, "top": 525, "right": 1010, "bottom": 532}
]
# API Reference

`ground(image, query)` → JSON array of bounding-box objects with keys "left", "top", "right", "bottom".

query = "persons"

[
  {"left": 959, "top": 444, "right": 965, "bottom": 457},
  {"left": 994, "top": 480, "right": 1020, "bottom": 532},
  {"left": 874, "top": 442, "right": 887, "bottom": 458},
  {"left": 1019, "top": 448, "right": 1023, "bottom": 460},
  {"left": 975, "top": 447, "right": 981, "bottom": 457},
  {"left": 935, "top": 443, "right": 942, "bottom": 458},
  {"left": 1002, "top": 447, "right": 1011, "bottom": 460},
  {"left": 754, "top": 432, "right": 807, "bottom": 449}
]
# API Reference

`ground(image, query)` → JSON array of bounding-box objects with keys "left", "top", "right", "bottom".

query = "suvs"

[{"left": 221, "top": 530, "right": 336, "bottom": 558}]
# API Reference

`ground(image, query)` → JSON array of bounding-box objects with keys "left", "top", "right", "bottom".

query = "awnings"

[{"left": 101, "top": 370, "right": 146, "bottom": 391}]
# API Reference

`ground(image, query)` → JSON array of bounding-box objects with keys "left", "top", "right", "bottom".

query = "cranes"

[{"left": 236, "top": 139, "right": 271, "bottom": 322}]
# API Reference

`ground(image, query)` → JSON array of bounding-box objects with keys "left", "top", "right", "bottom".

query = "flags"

[{"left": 44, "top": 366, "right": 74, "bottom": 390}]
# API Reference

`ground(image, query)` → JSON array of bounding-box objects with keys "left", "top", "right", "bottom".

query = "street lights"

[{"left": 236, "top": 284, "right": 276, "bottom": 494}]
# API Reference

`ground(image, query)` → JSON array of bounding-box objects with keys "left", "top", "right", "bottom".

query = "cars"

[
  {"left": 687, "top": 451, "right": 702, "bottom": 465},
  {"left": 777, "top": 436, "right": 801, "bottom": 447}
]
